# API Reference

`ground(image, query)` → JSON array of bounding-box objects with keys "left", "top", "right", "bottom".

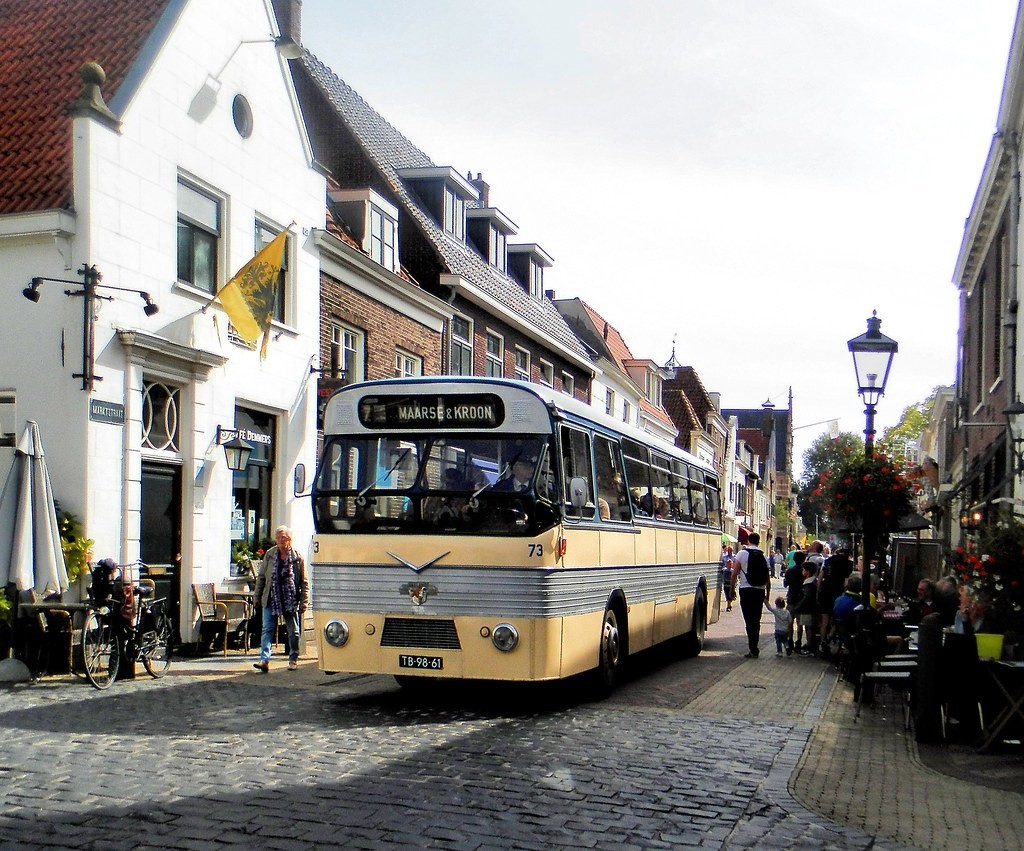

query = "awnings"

[
  {"left": 722, "top": 534, "right": 738, "bottom": 543},
  {"left": 739, "top": 527, "right": 752, "bottom": 542}
]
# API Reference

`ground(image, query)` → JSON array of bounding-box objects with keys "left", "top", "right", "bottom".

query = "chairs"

[
  {"left": 194, "top": 584, "right": 250, "bottom": 658},
  {"left": 20, "top": 588, "right": 91, "bottom": 679},
  {"left": 856, "top": 624, "right": 984, "bottom": 738}
]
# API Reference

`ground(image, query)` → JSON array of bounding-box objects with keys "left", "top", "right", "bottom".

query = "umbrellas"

[{"left": 0, "top": 420, "right": 70, "bottom": 599}]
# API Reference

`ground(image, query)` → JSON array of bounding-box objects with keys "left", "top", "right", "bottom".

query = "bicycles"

[{"left": 81, "top": 560, "right": 177, "bottom": 691}]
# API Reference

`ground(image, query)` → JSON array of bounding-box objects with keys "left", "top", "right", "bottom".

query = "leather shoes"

[
  {"left": 254, "top": 660, "right": 269, "bottom": 672},
  {"left": 287, "top": 661, "right": 298, "bottom": 670}
]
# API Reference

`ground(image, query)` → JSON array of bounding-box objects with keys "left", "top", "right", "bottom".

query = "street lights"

[{"left": 845, "top": 308, "right": 899, "bottom": 701}]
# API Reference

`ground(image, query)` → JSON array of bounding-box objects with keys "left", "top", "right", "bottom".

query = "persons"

[
  {"left": 585, "top": 472, "right": 671, "bottom": 521},
  {"left": 722, "top": 534, "right": 882, "bottom": 657},
  {"left": 426, "top": 451, "right": 549, "bottom": 523},
  {"left": 253, "top": 526, "right": 309, "bottom": 672},
  {"left": 906, "top": 578, "right": 990, "bottom": 638}
]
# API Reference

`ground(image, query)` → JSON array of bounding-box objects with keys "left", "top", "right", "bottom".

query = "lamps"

[
  {"left": 215, "top": 425, "right": 255, "bottom": 470},
  {"left": 96, "top": 284, "right": 159, "bottom": 317},
  {"left": 23, "top": 276, "right": 85, "bottom": 302},
  {"left": 1002, "top": 393, "right": 1024, "bottom": 473}
]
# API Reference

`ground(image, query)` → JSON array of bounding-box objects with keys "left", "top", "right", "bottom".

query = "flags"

[{"left": 220, "top": 233, "right": 286, "bottom": 361}]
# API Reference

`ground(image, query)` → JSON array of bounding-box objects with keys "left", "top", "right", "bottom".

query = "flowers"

[
  {"left": 257, "top": 549, "right": 265, "bottom": 557},
  {"left": 807, "top": 430, "right": 1017, "bottom": 622}
]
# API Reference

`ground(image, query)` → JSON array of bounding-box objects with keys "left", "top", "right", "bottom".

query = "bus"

[{"left": 292, "top": 376, "right": 726, "bottom": 702}]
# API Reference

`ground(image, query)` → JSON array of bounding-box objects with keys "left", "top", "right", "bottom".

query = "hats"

[
  {"left": 97, "top": 559, "right": 118, "bottom": 569},
  {"left": 518, "top": 449, "right": 540, "bottom": 470}
]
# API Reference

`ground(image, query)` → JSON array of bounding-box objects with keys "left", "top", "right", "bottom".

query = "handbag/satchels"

[
  {"left": 816, "top": 559, "right": 844, "bottom": 614},
  {"left": 119, "top": 564, "right": 136, "bottom": 619},
  {"left": 296, "top": 586, "right": 302, "bottom": 601},
  {"left": 784, "top": 579, "right": 787, "bottom": 587}
]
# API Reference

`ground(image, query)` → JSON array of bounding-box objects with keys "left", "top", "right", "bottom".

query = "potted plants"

[{"left": 234, "top": 541, "right": 253, "bottom": 575}]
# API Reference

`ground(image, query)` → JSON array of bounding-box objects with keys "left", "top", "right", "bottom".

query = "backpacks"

[{"left": 740, "top": 548, "right": 769, "bottom": 586}]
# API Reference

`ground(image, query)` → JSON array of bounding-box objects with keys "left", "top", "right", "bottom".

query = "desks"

[{"left": 979, "top": 659, "right": 1024, "bottom": 753}]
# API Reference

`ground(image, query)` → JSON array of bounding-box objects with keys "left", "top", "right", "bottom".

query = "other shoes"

[
  {"left": 727, "top": 607, "right": 730, "bottom": 611},
  {"left": 799, "top": 651, "right": 816, "bottom": 656},
  {"left": 794, "top": 647, "right": 800, "bottom": 653},
  {"left": 786, "top": 646, "right": 791, "bottom": 655},
  {"left": 745, "top": 652, "right": 758, "bottom": 657},
  {"left": 776, "top": 652, "right": 782, "bottom": 656},
  {"left": 753, "top": 649, "right": 759, "bottom": 654}
]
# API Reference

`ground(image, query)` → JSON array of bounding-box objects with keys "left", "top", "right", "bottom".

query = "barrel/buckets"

[{"left": 974, "top": 633, "right": 1004, "bottom": 661}]
